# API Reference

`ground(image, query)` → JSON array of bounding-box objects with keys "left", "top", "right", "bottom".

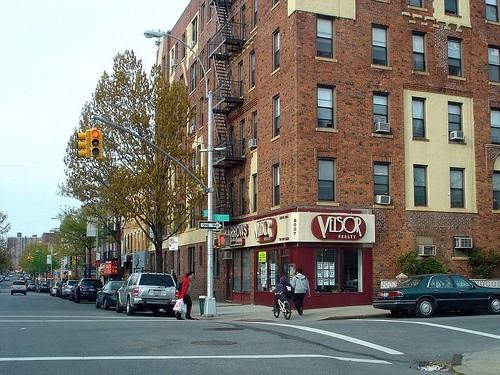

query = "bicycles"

[{"left": 270, "top": 290, "right": 292, "bottom": 320}]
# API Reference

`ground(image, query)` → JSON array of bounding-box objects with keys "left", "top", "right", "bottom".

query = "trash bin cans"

[{"left": 198, "top": 296, "right": 206, "bottom": 315}]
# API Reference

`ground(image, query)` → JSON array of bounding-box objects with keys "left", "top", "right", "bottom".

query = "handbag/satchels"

[{"left": 173, "top": 298, "right": 187, "bottom": 314}]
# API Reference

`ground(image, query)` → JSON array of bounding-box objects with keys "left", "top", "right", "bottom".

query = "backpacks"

[
  {"left": 175, "top": 282, "right": 182, "bottom": 293},
  {"left": 282, "top": 283, "right": 294, "bottom": 298}
]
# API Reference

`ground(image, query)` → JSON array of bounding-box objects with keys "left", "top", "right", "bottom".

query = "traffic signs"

[{"left": 198, "top": 220, "right": 224, "bottom": 230}]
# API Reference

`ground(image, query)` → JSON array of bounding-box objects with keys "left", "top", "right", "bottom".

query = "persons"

[
  {"left": 272, "top": 276, "right": 292, "bottom": 313},
  {"left": 292, "top": 268, "right": 311, "bottom": 315},
  {"left": 176, "top": 272, "right": 194, "bottom": 320}
]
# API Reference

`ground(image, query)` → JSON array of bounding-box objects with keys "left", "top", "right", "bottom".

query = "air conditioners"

[
  {"left": 418, "top": 244, "right": 437, "bottom": 256},
  {"left": 375, "top": 122, "right": 390, "bottom": 132},
  {"left": 450, "top": 130, "right": 464, "bottom": 140},
  {"left": 190, "top": 41, "right": 195, "bottom": 48},
  {"left": 221, "top": 250, "right": 232, "bottom": 260},
  {"left": 375, "top": 195, "right": 390, "bottom": 204},
  {"left": 171, "top": 58, "right": 178, "bottom": 67},
  {"left": 454, "top": 237, "right": 473, "bottom": 248},
  {"left": 190, "top": 125, "right": 196, "bottom": 134},
  {"left": 248, "top": 139, "right": 257, "bottom": 148}
]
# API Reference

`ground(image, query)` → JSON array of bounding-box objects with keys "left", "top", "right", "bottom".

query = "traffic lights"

[
  {"left": 90, "top": 130, "right": 101, "bottom": 157},
  {"left": 218, "top": 234, "right": 231, "bottom": 247},
  {"left": 76, "top": 129, "right": 90, "bottom": 159}
]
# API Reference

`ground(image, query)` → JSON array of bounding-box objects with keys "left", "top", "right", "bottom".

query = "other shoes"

[
  {"left": 186, "top": 317, "right": 194, "bottom": 320},
  {"left": 177, "top": 318, "right": 185, "bottom": 320},
  {"left": 298, "top": 307, "right": 303, "bottom": 315}
]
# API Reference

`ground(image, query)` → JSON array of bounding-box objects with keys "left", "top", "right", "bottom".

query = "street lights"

[{"left": 142, "top": 30, "right": 217, "bottom": 316}]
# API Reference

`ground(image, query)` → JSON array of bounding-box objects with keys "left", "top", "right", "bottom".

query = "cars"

[
  {"left": 61, "top": 280, "right": 79, "bottom": 298},
  {"left": 373, "top": 272, "right": 500, "bottom": 318},
  {"left": 51, "top": 282, "right": 61, "bottom": 296},
  {"left": 0, "top": 274, "right": 13, "bottom": 282},
  {"left": 17, "top": 273, "right": 50, "bottom": 293},
  {"left": 95, "top": 281, "right": 126, "bottom": 310}
]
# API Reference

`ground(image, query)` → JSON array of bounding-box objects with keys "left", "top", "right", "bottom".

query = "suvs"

[
  {"left": 116, "top": 272, "right": 180, "bottom": 316},
  {"left": 11, "top": 280, "right": 26, "bottom": 295},
  {"left": 73, "top": 278, "right": 104, "bottom": 303}
]
